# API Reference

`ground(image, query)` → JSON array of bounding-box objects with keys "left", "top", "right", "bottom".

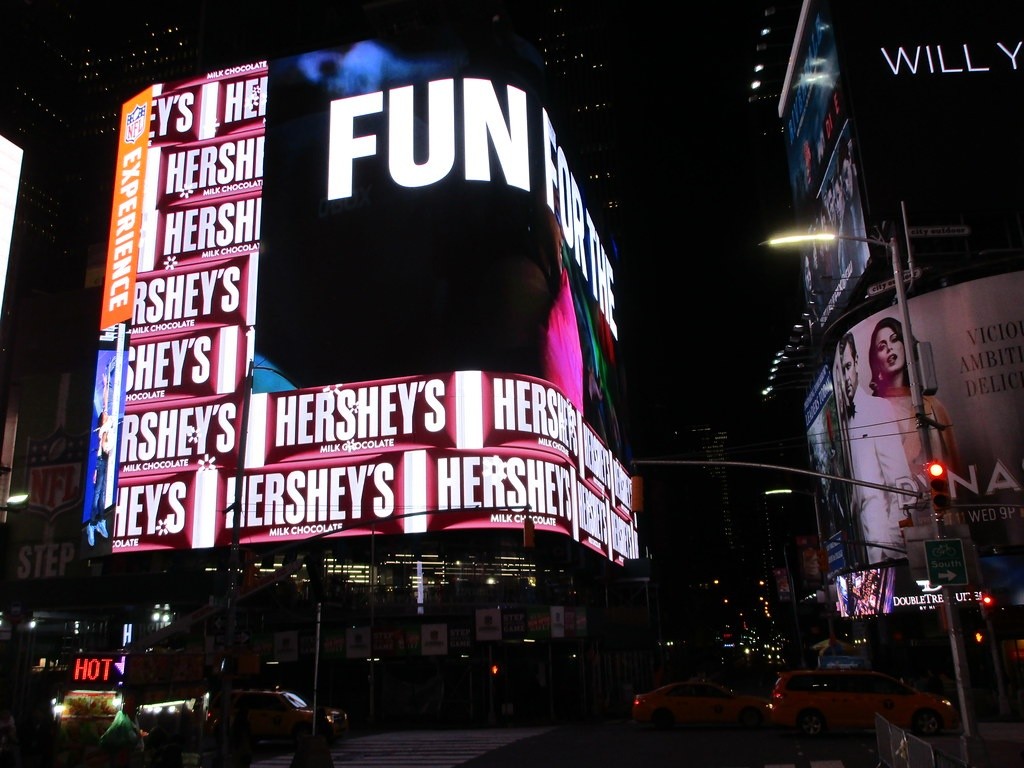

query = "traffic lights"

[
  {"left": 978, "top": 587, "right": 992, "bottom": 607},
  {"left": 927, "top": 465, "right": 953, "bottom": 514},
  {"left": 816, "top": 546, "right": 828, "bottom": 571}
]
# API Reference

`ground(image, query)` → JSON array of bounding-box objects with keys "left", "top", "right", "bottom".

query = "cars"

[{"left": 632, "top": 683, "right": 766, "bottom": 728}]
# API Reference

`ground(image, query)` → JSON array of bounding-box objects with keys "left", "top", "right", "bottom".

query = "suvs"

[
  {"left": 206, "top": 690, "right": 348, "bottom": 745},
  {"left": 767, "top": 668, "right": 961, "bottom": 736}
]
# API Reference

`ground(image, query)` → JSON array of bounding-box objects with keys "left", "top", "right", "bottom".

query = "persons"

[{"left": 838, "top": 318, "right": 966, "bottom": 564}]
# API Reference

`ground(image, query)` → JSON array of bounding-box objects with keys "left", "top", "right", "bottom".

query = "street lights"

[
  {"left": 765, "top": 226, "right": 993, "bottom": 767},
  {"left": 765, "top": 489, "right": 837, "bottom": 660}
]
